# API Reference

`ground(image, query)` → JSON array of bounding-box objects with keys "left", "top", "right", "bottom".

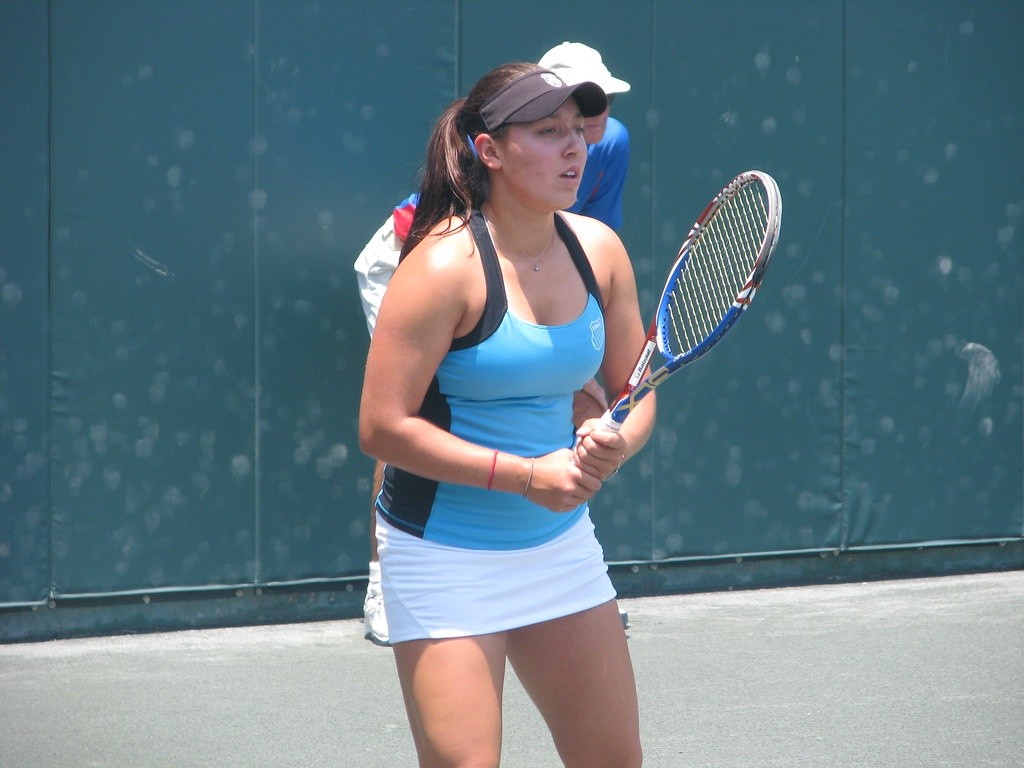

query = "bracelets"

[
  {"left": 604, "top": 454, "right": 624, "bottom": 482},
  {"left": 487, "top": 449, "right": 498, "bottom": 490},
  {"left": 523, "top": 457, "right": 535, "bottom": 497}
]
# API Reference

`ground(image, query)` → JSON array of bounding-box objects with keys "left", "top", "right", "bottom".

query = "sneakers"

[
  {"left": 364, "top": 583, "right": 388, "bottom": 642},
  {"left": 618, "top": 609, "right": 628, "bottom": 627}
]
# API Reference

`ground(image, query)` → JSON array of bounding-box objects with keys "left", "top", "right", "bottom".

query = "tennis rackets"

[{"left": 589, "top": 167, "right": 784, "bottom": 430}]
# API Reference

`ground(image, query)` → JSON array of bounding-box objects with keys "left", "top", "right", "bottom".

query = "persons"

[
  {"left": 357, "top": 62, "right": 658, "bottom": 768},
  {"left": 354, "top": 40, "right": 631, "bottom": 648}
]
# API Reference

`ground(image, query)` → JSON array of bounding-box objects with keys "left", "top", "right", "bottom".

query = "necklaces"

[{"left": 487, "top": 219, "right": 554, "bottom": 272}]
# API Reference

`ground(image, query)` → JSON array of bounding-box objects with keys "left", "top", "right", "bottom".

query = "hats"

[
  {"left": 537, "top": 42, "right": 630, "bottom": 95},
  {"left": 459, "top": 69, "right": 606, "bottom": 132}
]
